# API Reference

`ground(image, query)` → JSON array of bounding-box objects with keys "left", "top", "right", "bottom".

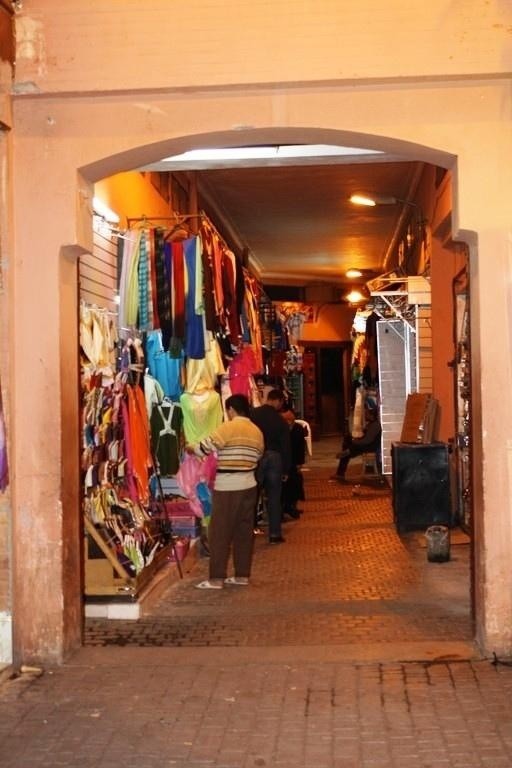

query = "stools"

[{"left": 359, "top": 452, "right": 385, "bottom": 484}]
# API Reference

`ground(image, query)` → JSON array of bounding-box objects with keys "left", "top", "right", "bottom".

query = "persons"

[
  {"left": 186, "top": 393, "right": 267, "bottom": 590},
  {"left": 249, "top": 389, "right": 291, "bottom": 544},
  {"left": 280, "top": 408, "right": 307, "bottom": 520},
  {"left": 331, "top": 409, "right": 382, "bottom": 480}
]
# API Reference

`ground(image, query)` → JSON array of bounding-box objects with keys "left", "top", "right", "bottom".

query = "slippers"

[
  {"left": 193, "top": 579, "right": 224, "bottom": 590},
  {"left": 224, "top": 576, "right": 249, "bottom": 585}
]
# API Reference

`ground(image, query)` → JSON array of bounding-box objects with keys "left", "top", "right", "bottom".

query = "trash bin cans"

[{"left": 427, "top": 526, "right": 448, "bottom": 559}]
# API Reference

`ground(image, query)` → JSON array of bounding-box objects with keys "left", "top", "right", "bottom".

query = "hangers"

[{"left": 164, "top": 210, "right": 199, "bottom": 243}]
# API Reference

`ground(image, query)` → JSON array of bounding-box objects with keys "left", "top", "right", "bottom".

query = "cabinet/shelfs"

[{"left": 390, "top": 440, "right": 451, "bottom": 535}]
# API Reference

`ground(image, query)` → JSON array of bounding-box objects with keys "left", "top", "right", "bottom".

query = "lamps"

[{"left": 349, "top": 189, "right": 427, "bottom": 230}]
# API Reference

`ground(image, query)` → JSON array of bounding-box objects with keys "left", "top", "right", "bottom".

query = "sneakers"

[
  {"left": 254, "top": 508, "right": 304, "bottom": 544},
  {"left": 336, "top": 448, "right": 350, "bottom": 460},
  {"left": 328, "top": 475, "right": 345, "bottom": 482}
]
